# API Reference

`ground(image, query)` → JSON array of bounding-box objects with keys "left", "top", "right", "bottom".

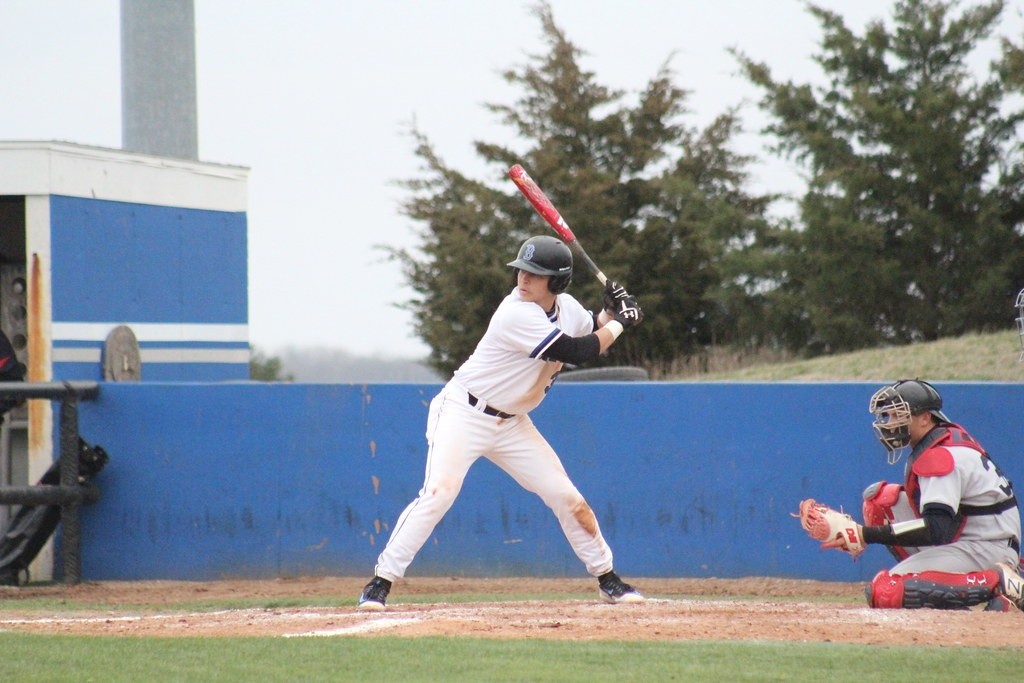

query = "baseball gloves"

[{"left": 799, "top": 498, "right": 863, "bottom": 556}]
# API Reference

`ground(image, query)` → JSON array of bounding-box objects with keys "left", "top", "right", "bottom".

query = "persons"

[
  {"left": 358, "top": 238, "right": 646, "bottom": 609},
  {"left": 798, "top": 379, "right": 1024, "bottom": 612}
]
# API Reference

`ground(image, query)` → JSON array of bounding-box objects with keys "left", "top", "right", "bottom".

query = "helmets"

[
  {"left": 507, "top": 235, "right": 573, "bottom": 294},
  {"left": 878, "top": 380, "right": 952, "bottom": 426}
]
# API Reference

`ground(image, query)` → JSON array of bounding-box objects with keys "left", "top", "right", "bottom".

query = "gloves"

[
  {"left": 602, "top": 281, "right": 628, "bottom": 316},
  {"left": 614, "top": 298, "right": 643, "bottom": 331}
]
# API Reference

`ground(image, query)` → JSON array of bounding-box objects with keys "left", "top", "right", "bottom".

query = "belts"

[
  {"left": 468, "top": 392, "right": 516, "bottom": 419},
  {"left": 1008, "top": 538, "right": 1020, "bottom": 559}
]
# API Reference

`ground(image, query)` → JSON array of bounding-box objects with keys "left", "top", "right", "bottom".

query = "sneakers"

[
  {"left": 357, "top": 580, "right": 390, "bottom": 612},
  {"left": 599, "top": 573, "right": 643, "bottom": 603},
  {"left": 994, "top": 556, "right": 1024, "bottom": 611}
]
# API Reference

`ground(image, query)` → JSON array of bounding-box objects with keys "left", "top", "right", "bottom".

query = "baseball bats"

[{"left": 507, "top": 163, "right": 607, "bottom": 286}]
